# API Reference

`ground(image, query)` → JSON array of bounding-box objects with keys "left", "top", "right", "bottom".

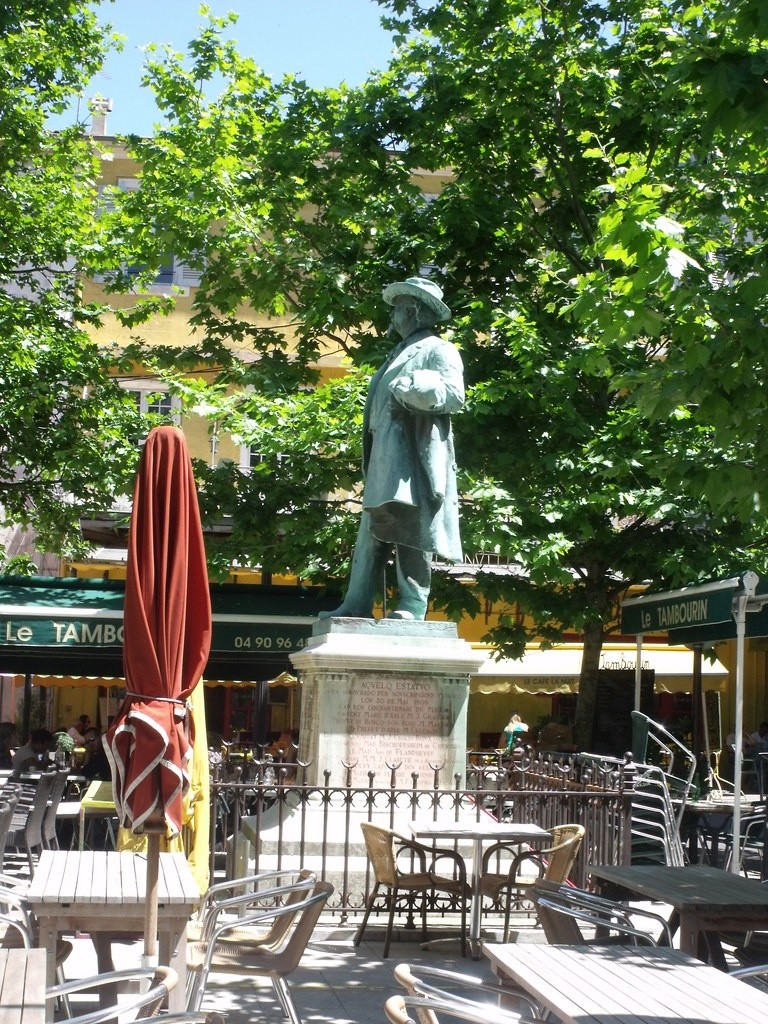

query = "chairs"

[{"left": 0, "top": 710, "right": 767, "bottom": 1024}]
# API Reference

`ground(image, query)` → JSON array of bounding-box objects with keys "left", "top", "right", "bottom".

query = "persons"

[
  {"left": 0, "top": 713, "right": 114, "bottom": 780},
  {"left": 500, "top": 712, "right": 768, "bottom": 785},
  {"left": 321, "top": 279, "right": 466, "bottom": 619}
]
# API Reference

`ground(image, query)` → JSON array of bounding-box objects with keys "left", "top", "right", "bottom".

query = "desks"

[
  {"left": 409, "top": 820, "right": 553, "bottom": 962},
  {"left": 78, "top": 780, "right": 116, "bottom": 850},
  {"left": 671, "top": 799, "right": 755, "bottom": 867},
  {"left": 27, "top": 849, "right": 200, "bottom": 1024},
  {"left": 1, "top": 769, "right": 86, "bottom": 781},
  {"left": 585, "top": 865, "right": 767, "bottom": 962},
  {"left": 482, "top": 943, "right": 767, "bottom": 1024},
  {"left": 231, "top": 780, "right": 289, "bottom": 797}
]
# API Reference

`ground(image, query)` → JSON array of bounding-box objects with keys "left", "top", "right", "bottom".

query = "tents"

[{"left": 620, "top": 570, "right": 768, "bottom": 875}]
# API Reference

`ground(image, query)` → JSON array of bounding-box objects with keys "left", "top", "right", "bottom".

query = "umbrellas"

[{"left": 104, "top": 427, "right": 214, "bottom": 968}]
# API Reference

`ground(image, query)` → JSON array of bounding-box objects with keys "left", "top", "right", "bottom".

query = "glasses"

[
  {"left": 84, "top": 734, "right": 100, "bottom": 745},
  {"left": 84, "top": 720, "right": 90, "bottom": 724}
]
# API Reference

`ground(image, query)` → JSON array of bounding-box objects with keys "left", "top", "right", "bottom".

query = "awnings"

[
  {"left": 0, "top": 565, "right": 375, "bottom": 685},
  {"left": 461, "top": 643, "right": 732, "bottom": 692}
]
